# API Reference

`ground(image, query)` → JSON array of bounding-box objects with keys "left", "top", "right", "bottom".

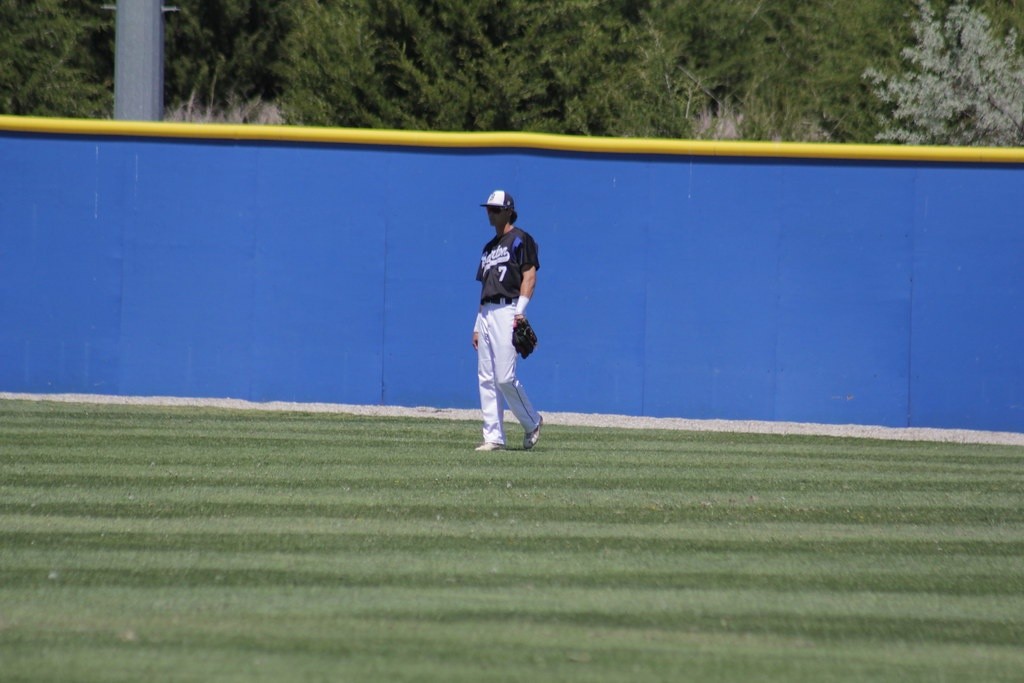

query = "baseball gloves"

[{"left": 511, "top": 318, "right": 537, "bottom": 360}]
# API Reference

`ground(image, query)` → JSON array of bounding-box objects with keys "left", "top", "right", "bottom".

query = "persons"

[{"left": 471, "top": 190, "right": 543, "bottom": 450}]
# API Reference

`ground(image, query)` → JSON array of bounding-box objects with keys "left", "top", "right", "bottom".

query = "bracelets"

[
  {"left": 515, "top": 295, "right": 530, "bottom": 317},
  {"left": 473, "top": 312, "right": 481, "bottom": 332}
]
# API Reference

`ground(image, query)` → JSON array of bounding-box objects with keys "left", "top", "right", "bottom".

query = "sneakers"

[
  {"left": 523, "top": 416, "right": 543, "bottom": 448},
  {"left": 475, "top": 442, "right": 506, "bottom": 451}
]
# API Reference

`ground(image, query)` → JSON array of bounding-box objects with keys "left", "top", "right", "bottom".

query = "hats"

[{"left": 480, "top": 190, "right": 514, "bottom": 208}]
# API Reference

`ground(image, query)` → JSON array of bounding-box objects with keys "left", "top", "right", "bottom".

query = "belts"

[{"left": 483, "top": 296, "right": 513, "bottom": 305}]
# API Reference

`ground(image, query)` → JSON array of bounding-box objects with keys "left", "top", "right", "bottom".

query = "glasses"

[{"left": 487, "top": 206, "right": 510, "bottom": 214}]
